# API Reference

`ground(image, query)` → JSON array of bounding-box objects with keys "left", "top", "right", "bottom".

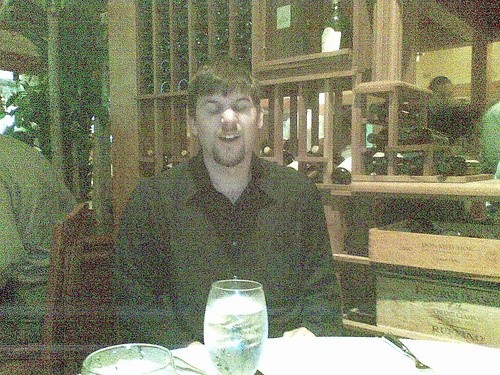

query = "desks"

[{"left": 169, "top": 336, "right": 500, "bottom": 375}]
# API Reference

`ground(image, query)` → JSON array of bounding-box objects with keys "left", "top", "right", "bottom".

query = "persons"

[
  {"left": 116, "top": 55, "right": 345, "bottom": 351},
  {"left": 0, "top": 132, "right": 78, "bottom": 323},
  {"left": 429, "top": 75, "right": 455, "bottom": 97}
]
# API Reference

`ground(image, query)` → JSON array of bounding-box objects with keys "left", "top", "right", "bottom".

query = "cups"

[
  {"left": 204, "top": 278, "right": 270, "bottom": 375},
  {"left": 81, "top": 343, "right": 176, "bottom": 375}
]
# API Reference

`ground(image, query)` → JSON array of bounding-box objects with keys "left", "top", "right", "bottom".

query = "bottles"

[
  {"left": 138, "top": 2, "right": 253, "bottom": 96},
  {"left": 284, "top": 101, "right": 483, "bottom": 185},
  {"left": 145, "top": 144, "right": 198, "bottom": 179},
  {"left": 320, "top": 1, "right": 351, "bottom": 51}
]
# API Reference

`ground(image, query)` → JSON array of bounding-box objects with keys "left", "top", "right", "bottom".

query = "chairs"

[{"left": 0, "top": 201, "right": 90, "bottom": 375}]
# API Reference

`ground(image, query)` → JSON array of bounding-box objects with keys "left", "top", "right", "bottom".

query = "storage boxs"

[{"left": 369, "top": 218, "right": 500, "bottom": 348}]
